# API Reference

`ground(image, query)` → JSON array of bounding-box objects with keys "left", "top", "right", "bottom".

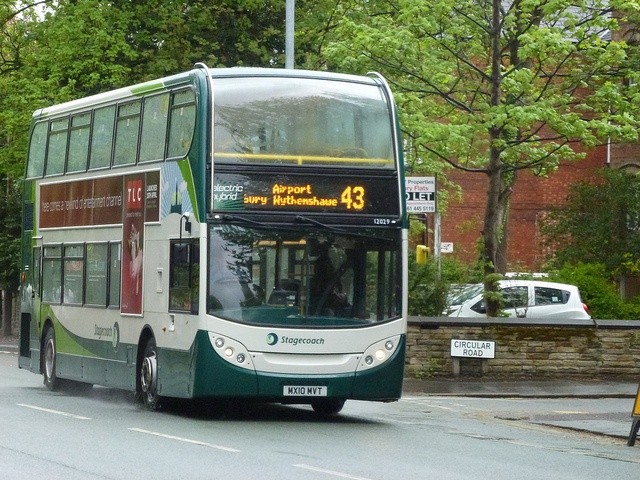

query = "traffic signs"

[{"left": 451, "top": 339, "right": 495, "bottom": 360}]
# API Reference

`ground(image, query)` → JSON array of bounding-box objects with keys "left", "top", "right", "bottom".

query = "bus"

[{"left": 17, "top": 61, "right": 409, "bottom": 412}]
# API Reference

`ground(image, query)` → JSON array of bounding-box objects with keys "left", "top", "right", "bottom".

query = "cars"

[
  {"left": 449, "top": 280, "right": 593, "bottom": 319},
  {"left": 439, "top": 285, "right": 485, "bottom": 317}
]
